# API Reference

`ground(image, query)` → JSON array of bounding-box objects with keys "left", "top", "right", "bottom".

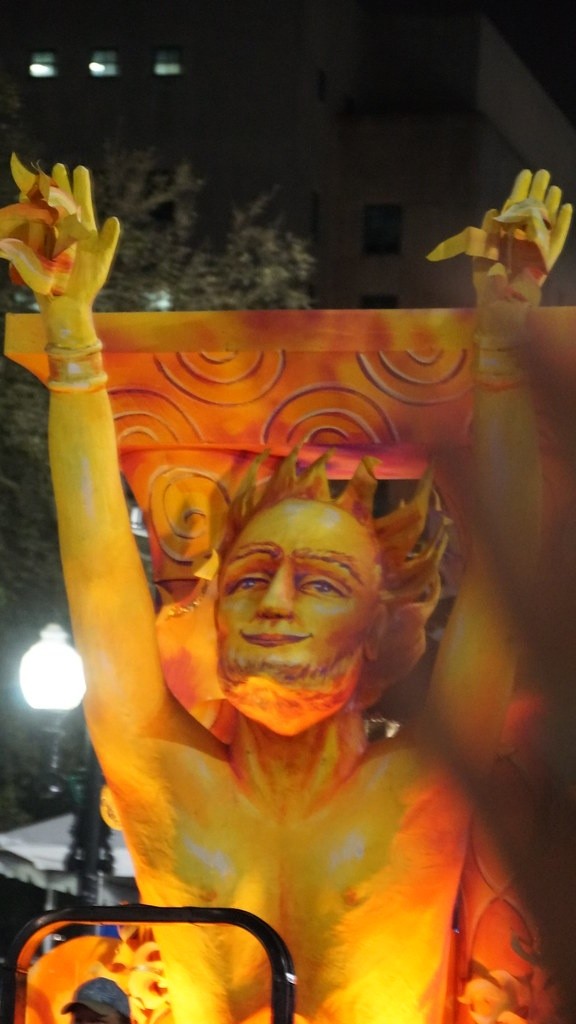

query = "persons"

[
  {"left": 61, "top": 977, "right": 138, "bottom": 1024},
  {"left": 0, "top": 161, "right": 576, "bottom": 1024}
]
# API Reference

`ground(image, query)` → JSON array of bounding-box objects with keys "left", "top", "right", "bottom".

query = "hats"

[{"left": 61, "top": 977, "right": 130, "bottom": 1017}]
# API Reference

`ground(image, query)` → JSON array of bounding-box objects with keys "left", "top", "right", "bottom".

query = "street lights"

[{"left": 16, "top": 622, "right": 131, "bottom": 910}]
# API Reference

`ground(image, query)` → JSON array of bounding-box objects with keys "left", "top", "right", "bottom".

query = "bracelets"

[
  {"left": 45, "top": 373, "right": 109, "bottom": 394},
  {"left": 43, "top": 340, "right": 101, "bottom": 356},
  {"left": 467, "top": 362, "right": 533, "bottom": 390},
  {"left": 469, "top": 327, "right": 532, "bottom": 351}
]
